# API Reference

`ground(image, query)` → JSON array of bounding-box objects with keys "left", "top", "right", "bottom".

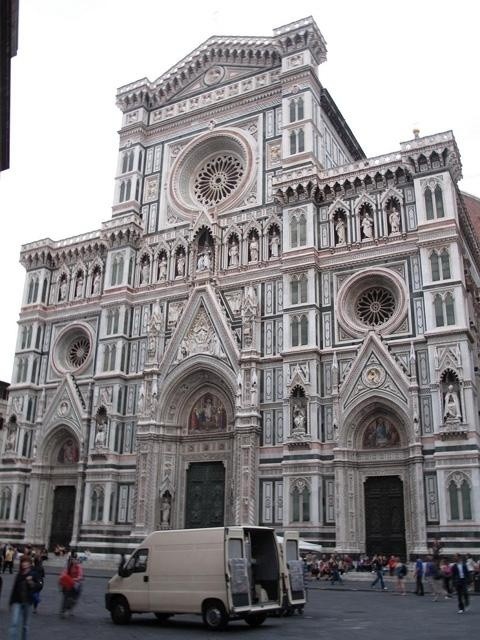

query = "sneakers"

[{"left": 369, "top": 586, "right": 471, "bottom": 614}]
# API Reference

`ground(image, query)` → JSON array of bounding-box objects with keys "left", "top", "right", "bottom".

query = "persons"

[
  {"left": 450, "top": 552, "right": 473, "bottom": 615},
  {"left": 300, "top": 548, "right": 480, "bottom": 602},
  {"left": 157, "top": 256, "right": 166, "bottom": 280},
  {"left": 6, "top": 555, "right": 43, "bottom": 640},
  {"left": 176, "top": 254, "right": 185, "bottom": 280},
  {"left": 291, "top": 397, "right": 307, "bottom": 428},
  {"left": 195, "top": 241, "right": 215, "bottom": 273},
  {"left": 162, "top": 497, "right": 170, "bottom": 523},
  {"left": 334, "top": 216, "right": 348, "bottom": 245},
  {"left": 76, "top": 277, "right": 84, "bottom": 297},
  {"left": 440, "top": 382, "right": 462, "bottom": 424},
  {"left": 1, "top": 538, "right": 91, "bottom": 619},
  {"left": 388, "top": 205, "right": 401, "bottom": 234},
  {"left": 360, "top": 212, "right": 374, "bottom": 239},
  {"left": 96, "top": 416, "right": 108, "bottom": 446},
  {"left": 140, "top": 260, "right": 150, "bottom": 286},
  {"left": 117, "top": 552, "right": 125, "bottom": 567},
  {"left": 92, "top": 269, "right": 101, "bottom": 294},
  {"left": 228, "top": 241, "right": 240, "bottom": 268},
  {"left": 269, "top": 232, "right": 281, "bottom": 257},
  {"left": 59, "top": 278, "right": 67, "bottom": 303},
  {"left": 248, "top": 236, "right": 259, "bottom": 263}
]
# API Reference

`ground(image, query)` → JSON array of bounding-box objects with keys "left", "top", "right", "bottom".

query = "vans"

[{"left": 101, "top": 520, "right": 305, "bottom": 628}]
